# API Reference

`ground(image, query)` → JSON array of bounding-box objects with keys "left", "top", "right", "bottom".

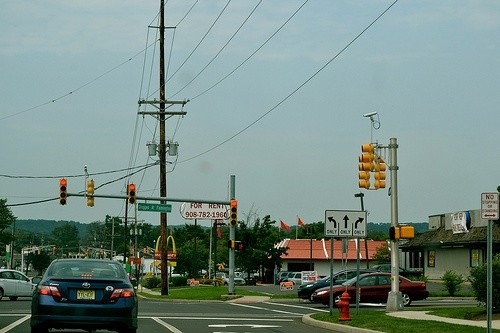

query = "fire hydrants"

[{"left": 337, "top": 292, "right": 352, "bottom": 321}]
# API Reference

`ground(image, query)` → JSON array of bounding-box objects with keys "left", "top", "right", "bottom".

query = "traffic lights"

[
  {"left": 60, "top": 178, "right": 67, "bottom": 206},
  {"left": 235, "top": 241, "right": 243, "bottom": 252},
  {"left": 361, "top": 144, "right": 375, "bottom": 171},
  {"left": 230, "top": 199, "right": 238, "bottom": 227},
  {"left": 128, "top": 183, "right": 136, "bottom": 204},
  {"left": 375, "top": 163, "right": 386, "bottom": 188}
]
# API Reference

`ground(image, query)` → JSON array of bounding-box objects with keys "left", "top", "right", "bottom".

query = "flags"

[
  {"left": 298, "top": 218, "right": 304, "bottom": 226},
  {"left": 281, "top": 221, "right": 290, "bottom": 231}
]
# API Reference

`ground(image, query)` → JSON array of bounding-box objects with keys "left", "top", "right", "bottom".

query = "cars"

[
  {"left": 30, "top": 258, "right": 138, "bottom": 333},
  {"left": 371, "top": 264, "right": 422, "bottom": 277},
  {"left": 298, "top": 269, "right": 378, "bottom": 303},
  {"left": 278, "top": 271, "right": 326, "bottom": 285},
  {"left": 311, "top": 272, "right": 429, "bottom": 307},
  {"left": 0, "top": 268, "right": 37, "bottom": 301},
  {"left": 215, "top": 268, "right": 265, "bottom": 286}
]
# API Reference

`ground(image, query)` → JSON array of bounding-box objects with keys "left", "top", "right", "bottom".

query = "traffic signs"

[{"left": 324, "top": 210, "right": 367, "bottom": 238}]
